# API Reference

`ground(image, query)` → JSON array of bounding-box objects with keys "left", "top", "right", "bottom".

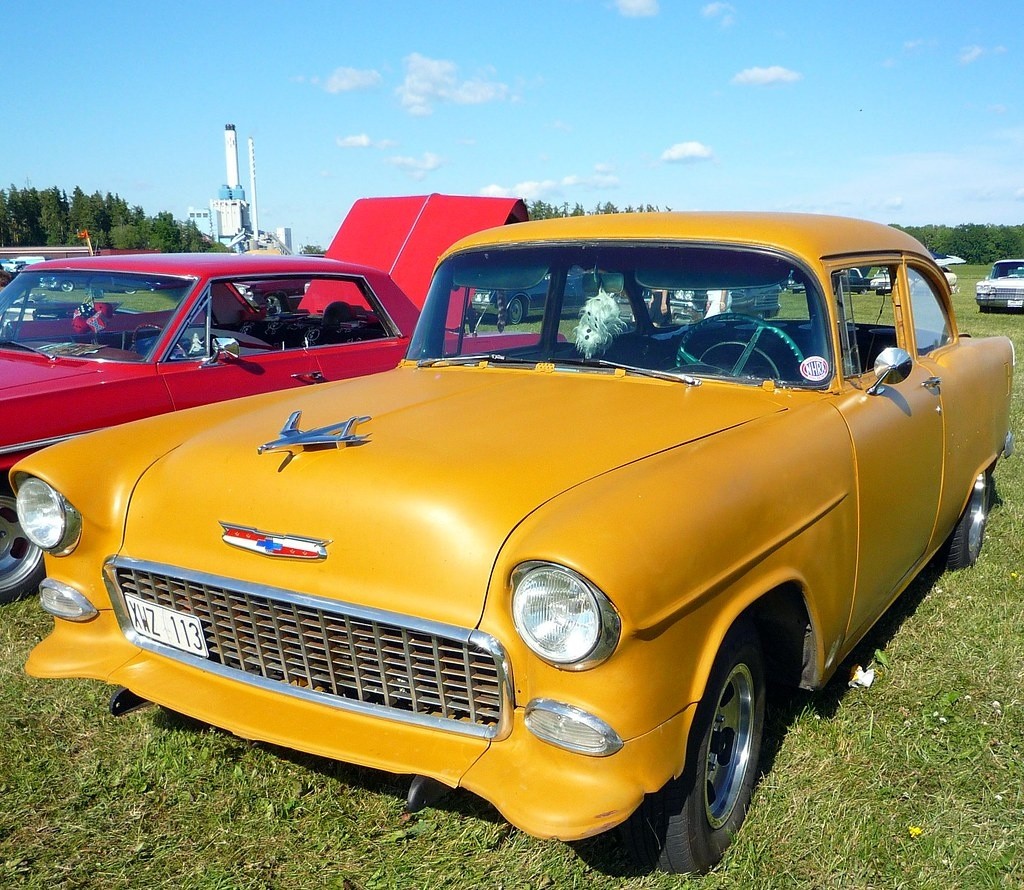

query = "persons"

[
  {"left": 648, "top": 291, "right": 672, "bottom": 326},
  {"left": 703, "top": 290, "right": 733, "bottom": 319}
]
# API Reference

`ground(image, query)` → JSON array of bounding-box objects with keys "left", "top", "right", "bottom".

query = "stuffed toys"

[{"left": 574, "top": 292, "right": 623, "bottom": 359}]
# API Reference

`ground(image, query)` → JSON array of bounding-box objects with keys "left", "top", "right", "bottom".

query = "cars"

[
  {"left": 869, "top": 264, "right": 957, "bottom": 293},
  {"left": 670, "top": 284, "right": 783, "bottom": 325},
  {"left": 0, "top": 191, "right": 569, "bottom": 606},
  {"left": 975, "top": 259, "right": 1024, "bottom": 313},
  {"left": 470, "top": 259, "right": 590, "bottom": 327},
  {"left": 8, "top": 210, "right": 1018, "bottom": 874},
  {"left": 784, "top": 270, "right": 875, "bottom": 295}
]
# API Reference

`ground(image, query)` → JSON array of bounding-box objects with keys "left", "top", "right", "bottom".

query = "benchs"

[{"left": 230, "top": 291, "right": 388, "bottom": 347}]
